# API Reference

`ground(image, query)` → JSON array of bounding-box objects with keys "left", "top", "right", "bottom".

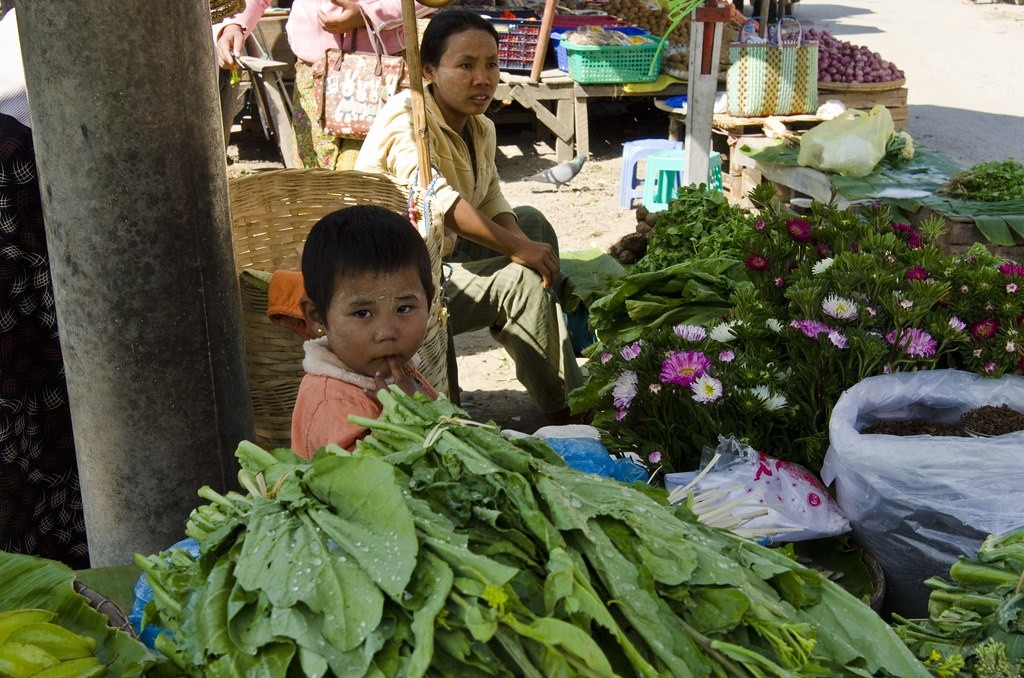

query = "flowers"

[{"left": 564, "top": 174, "right": 1024, "bottom": 487}]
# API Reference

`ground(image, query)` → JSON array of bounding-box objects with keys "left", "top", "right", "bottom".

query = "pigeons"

[{"left": 522, "top": 155, "right": 588, "bottom": 189}]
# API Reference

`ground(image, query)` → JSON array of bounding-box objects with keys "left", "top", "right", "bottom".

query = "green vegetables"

[
  {"left": 140, "top": 380, "right": 1024, "bottom": 678},
  {"left": 638, "top": 182, "right": 742, "bottom": 272},
  {"left": 938, "top": 152, "right": 1020, "bottom": 203}
]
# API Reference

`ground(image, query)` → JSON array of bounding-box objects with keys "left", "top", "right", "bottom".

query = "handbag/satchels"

[
  {"left": 728, "top": 16, "right": 820, "bottom": 115},
  {"left": 313, "top": 5, "right": 407, "bottom": 139}
]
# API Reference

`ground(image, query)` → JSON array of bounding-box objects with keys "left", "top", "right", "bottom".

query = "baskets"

[
  {"left": 444, "top": 7, "right": 666, "bottom": 84},
  {"left": 226, "top": 167, "right": 451, "bottom": 465}
]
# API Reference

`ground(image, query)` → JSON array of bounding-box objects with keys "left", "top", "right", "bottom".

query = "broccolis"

[{"left": 888, "top": 131, "right": 916, "bottom": 160}]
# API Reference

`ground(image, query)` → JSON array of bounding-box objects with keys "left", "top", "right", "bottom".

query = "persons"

[
  {"left": 356, "top": 8, "right": 601, "bottom": 426},
  {"left": 289, "top": 204, "right": 443, "bottom": 458},
  {"left": 212, "top": 0, "right": 442, "bottom": 167}
]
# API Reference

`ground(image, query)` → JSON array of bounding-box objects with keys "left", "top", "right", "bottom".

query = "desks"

[
  {"left": 733, "top": 133, "right": 969, "bottom": 217},
  {"left": 573, "top": 81, "right": 686, "bottom": 159},
  {"left": 669, "top": 108, "right": 834, "bottom": 177},
  {"left": 494, "top": 82, "right": 573, "bottom": 164}
]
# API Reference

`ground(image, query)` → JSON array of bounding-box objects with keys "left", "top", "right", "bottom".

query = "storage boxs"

[
  {"left": 476, "top": 8, "right": 543, "bottom": 72},
  {"left": 818, "top": 90, "right": 907, "bottom": 135},
  {"left": 559, "top": 34, "right": 670, "bottom": 83},
  {"left": 549, "top": 25, "right": 651, "bottom": 74},
  {"left": 248, "top": 17, "right": 298, "bottom": 81},
  {"left": 552, "top": 15, "right": 627, "bottom": 28}
]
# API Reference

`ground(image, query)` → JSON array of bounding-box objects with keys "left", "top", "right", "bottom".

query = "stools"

[
  {"left": 621, "top": 138, "right": 685, "bottom": 212},
  {"left": 219, "top": 53, "right": 305, "bottom": 170},
  {"left": 643, "top": 150, "right": 724, "bottom": 214}
]
